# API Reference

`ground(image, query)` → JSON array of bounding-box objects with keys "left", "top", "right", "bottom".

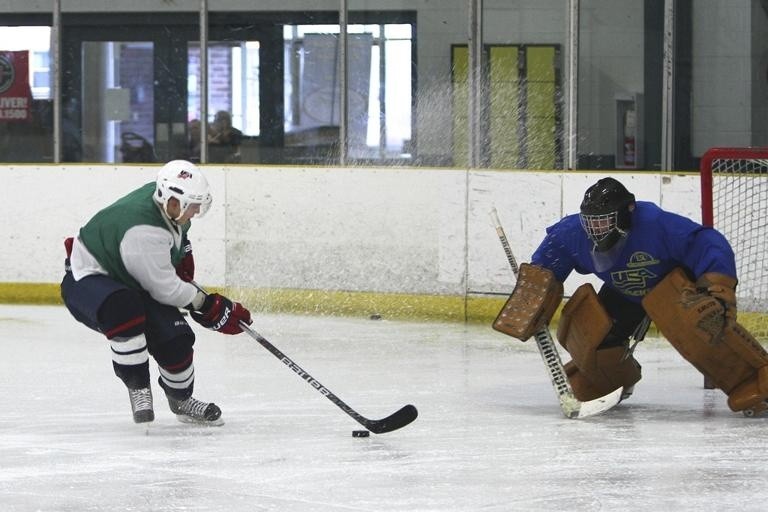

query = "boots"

[
  {"left": 167, "top": 397, "right": 221, "bottom": 420},
  {"left": 128, "top": 387, "right": 154, "bottom": 423}
]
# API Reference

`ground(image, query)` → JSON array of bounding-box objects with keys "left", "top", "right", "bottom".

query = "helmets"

[
  {"left": 578, "top": 178, "right": 635, "bottom": 250},
  {"left": 153, "top": 160, "right": 213, "bottom": 222}
]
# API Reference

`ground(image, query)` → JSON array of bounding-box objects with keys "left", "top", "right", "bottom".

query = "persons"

[
  {"left": 208, "top": 109, "right": 244, "bottom": 147},
  {"left": 490, "top": 177, "right": 768, "bottom": 416},
  {"left": 59, "top": 158, "right": 253, "bottom": 422}
]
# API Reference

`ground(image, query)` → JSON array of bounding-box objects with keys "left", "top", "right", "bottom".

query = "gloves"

[
  {"left": 192, "top": 294, "right": 250, "bottom": 334},
  {"left": 178, "top": 241, "right": 194, "bottom": 282}
]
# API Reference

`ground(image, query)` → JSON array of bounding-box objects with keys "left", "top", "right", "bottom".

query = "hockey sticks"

[
  {"left": 190, "top": 281, "right": 418, "bottom": 434},
  {"left": 489, "top": 205, "right": 624, "bottom": 417}
]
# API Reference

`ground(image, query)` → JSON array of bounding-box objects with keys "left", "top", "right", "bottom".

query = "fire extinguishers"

[{"left": 625, "top": 133, "right": 634, "bottom": 165}]
